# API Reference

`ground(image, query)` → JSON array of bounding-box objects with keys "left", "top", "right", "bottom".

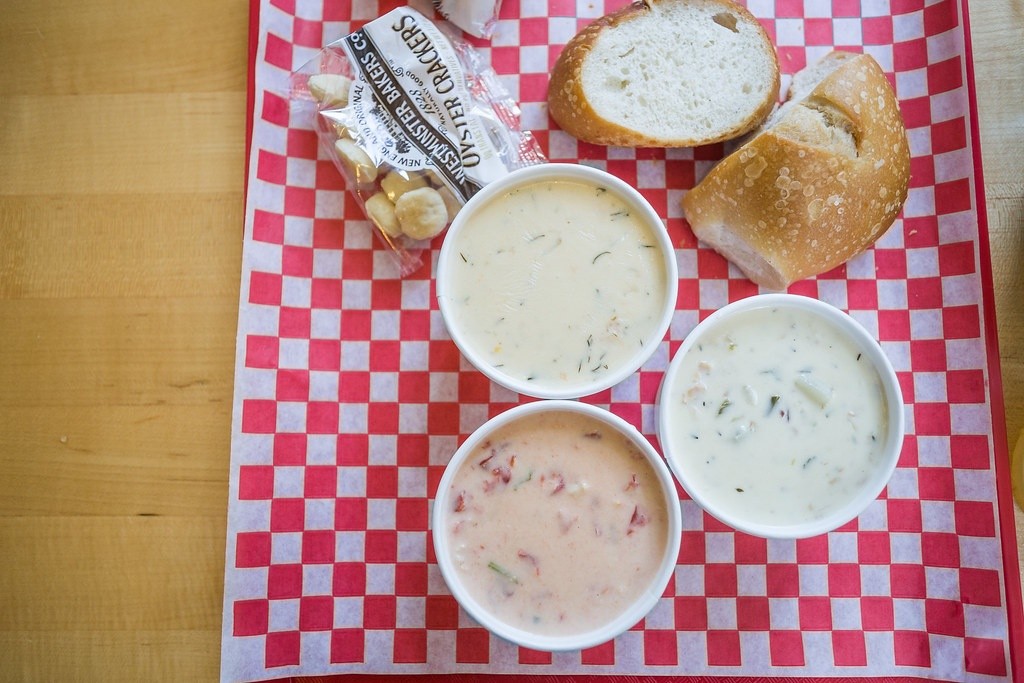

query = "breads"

[
  {"left": 547, "top": 1, "right": 781, "bottom": 148},
  {"left": 681, "top": 52, "right": 911, "bottom": 288}
]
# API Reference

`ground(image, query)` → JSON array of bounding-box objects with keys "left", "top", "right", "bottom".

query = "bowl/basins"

[
  {"left": 654, "top": 293, "right": 905, "bottom": 540},
  {"left": 432, "top": 400, "right": 682, "bottom": 652},
  {"left": 435, "top": 162, "right": 678, "bottom": 399}
]
